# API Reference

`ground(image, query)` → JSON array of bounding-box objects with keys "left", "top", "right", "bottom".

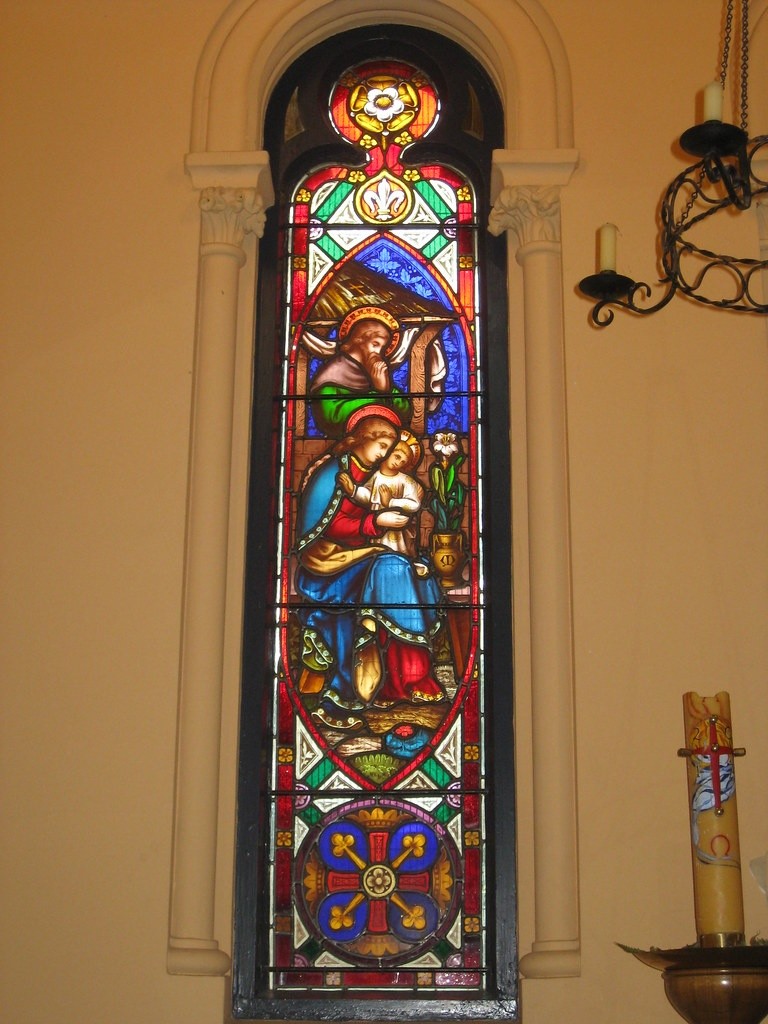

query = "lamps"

[{"left": 579, "top": 0, "right": 768, "bottom": 326}]
